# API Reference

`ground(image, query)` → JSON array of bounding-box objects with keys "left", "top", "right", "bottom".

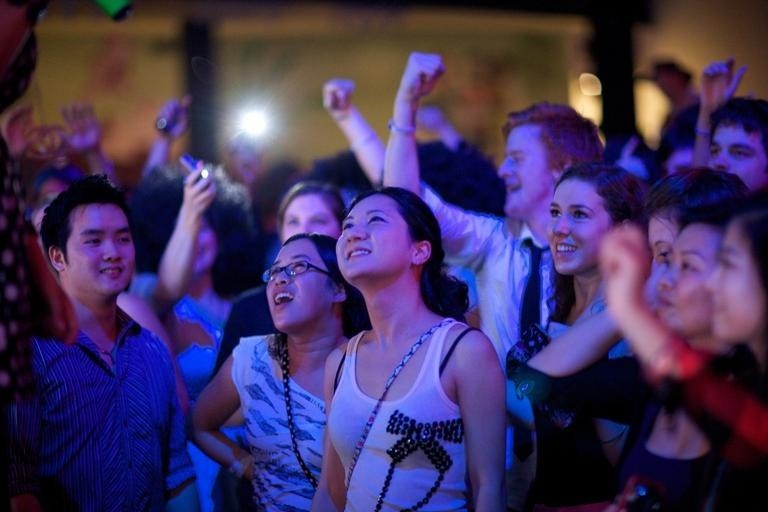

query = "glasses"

[{"left": 262, "top": 261, "right": 334, "bottom": 283}]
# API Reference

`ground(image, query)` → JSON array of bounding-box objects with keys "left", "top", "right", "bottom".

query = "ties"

[{"left": 513, "top": 238, "right": 550, "bottom": 462}]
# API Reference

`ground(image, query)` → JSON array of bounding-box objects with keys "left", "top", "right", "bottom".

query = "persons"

[{"left": 0, "top": 52, "right": 766, "bottom": 510}]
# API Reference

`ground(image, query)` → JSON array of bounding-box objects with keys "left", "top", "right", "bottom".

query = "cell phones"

[{"left": 179, "top": 152, "right": 206, "bottom": 180}]
